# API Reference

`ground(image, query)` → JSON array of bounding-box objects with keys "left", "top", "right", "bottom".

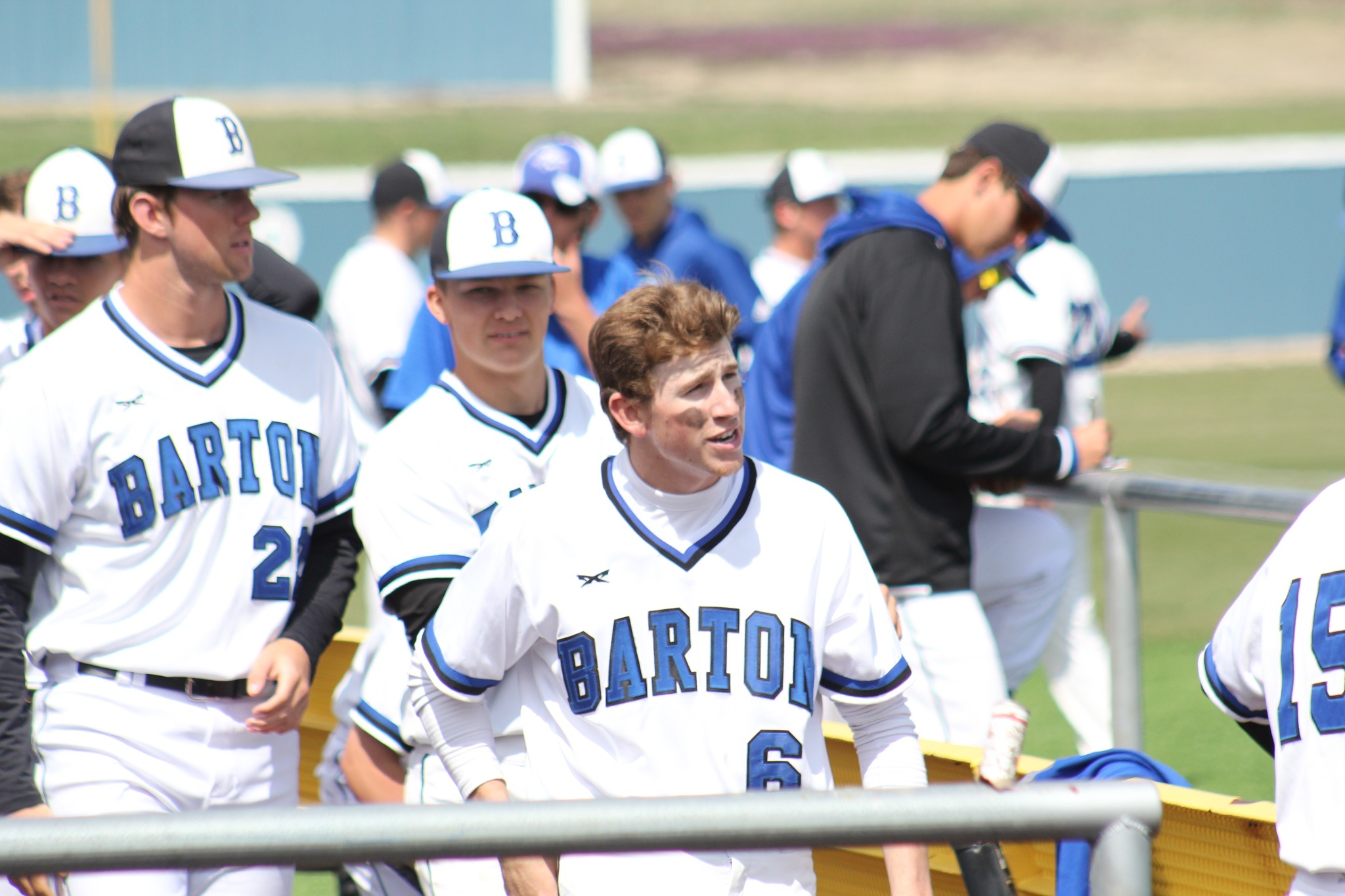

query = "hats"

[
  {"left": 970, "top": 124, "right": 1074, "bottom": 243},
  {"left": 430, "top": 187, "right": 572, "bottom": 279},
  {"left": 113, "top": 97, "right": 297, "bottom": 190},
  {"left": 22, "top": 146, "right": 129, "bottom": 256},
  {"left": 597, "top": 128, "right": 665, "bottom": 191},
  {"left": 520, "top": 140, "right": 597, "bottom": 205},
  {"left": 372, "top": 149, "right": 469, "bottom": 210},
  {"left": 765, "top": 146, "right": 852, "bottom": 206}
]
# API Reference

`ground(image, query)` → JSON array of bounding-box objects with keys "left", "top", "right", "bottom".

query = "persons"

[
  {"left": 0, "top": 98, "right": 1148, "bottom": 896},
  {"left": 1198, "top": 479, "right": 1345, "bottom": 896}
]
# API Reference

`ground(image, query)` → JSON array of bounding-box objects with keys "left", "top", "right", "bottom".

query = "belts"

[{"left": 39, "top": 655, "right": 257, "bottom": 702}]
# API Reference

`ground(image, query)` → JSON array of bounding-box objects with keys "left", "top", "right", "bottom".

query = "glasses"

[{"left": 529, "top": 191, "right": 581, "bottom": 217}]
